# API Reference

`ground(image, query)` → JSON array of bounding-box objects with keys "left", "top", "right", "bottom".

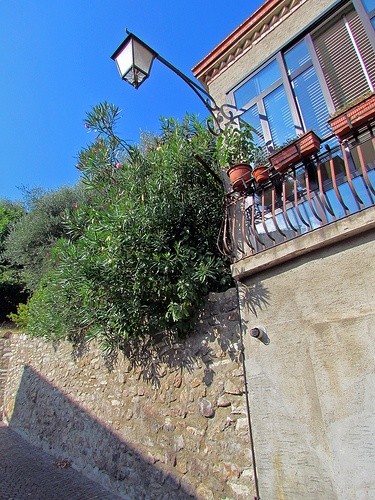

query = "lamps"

[{"left": 111, "top": 28, "right": 223, "bottom": 137}]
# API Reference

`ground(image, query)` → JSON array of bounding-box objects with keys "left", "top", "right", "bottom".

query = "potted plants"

[
  {"left": 327, "top": 89, "right": 375, "bottom": 141},
  {"left": 212, "top": 122, "right": 254, "bottom": 191},
  {"left": 268, "top": 130, "right": 322, "bottom": 173},
  {"left": 252, "top": 146, "right": 269, "bottom": 183}
]
